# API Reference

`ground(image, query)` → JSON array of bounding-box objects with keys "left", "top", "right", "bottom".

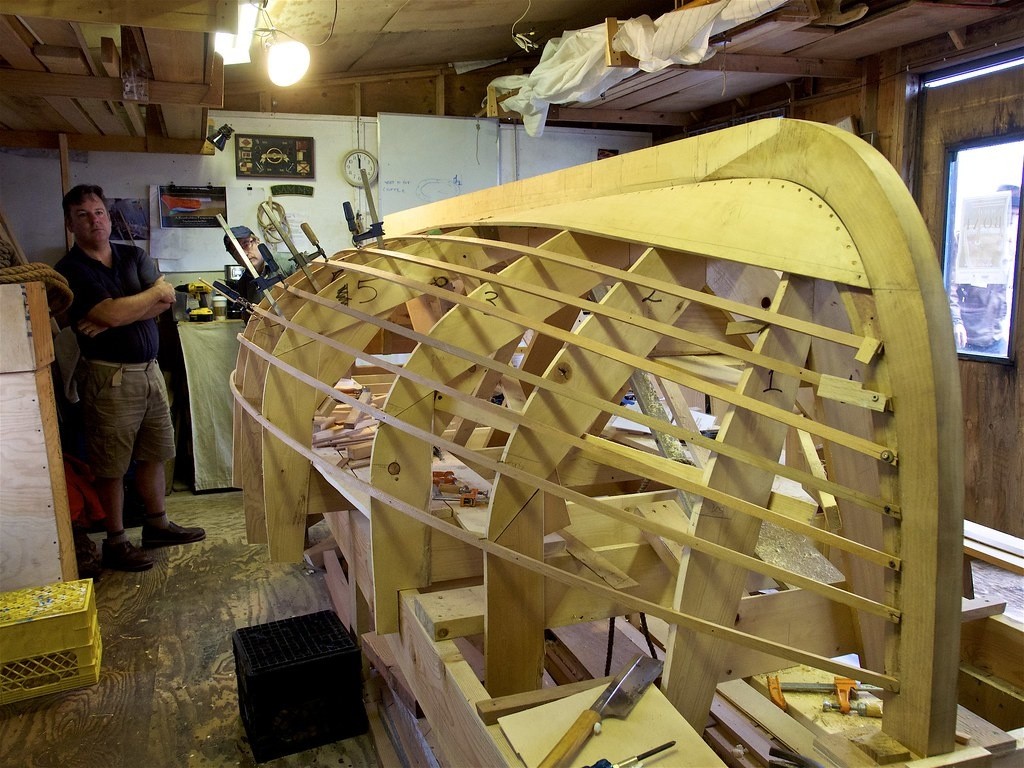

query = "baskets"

[
  {"left": 0, "top": 576, "right": 104, "bottom": 708},
  {"left": 230, "top": 609, "right": 370, "bottom": 764}
]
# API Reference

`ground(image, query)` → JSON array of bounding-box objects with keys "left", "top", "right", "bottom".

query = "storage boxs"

[
  {"left": 232, "top": 611, "right": 369, "bottom": 763},
  {"left": 0, "top": 578, "right": 108, "bottom": 707}
]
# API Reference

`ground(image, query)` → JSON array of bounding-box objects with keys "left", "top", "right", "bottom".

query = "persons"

[
  {"left": 48, "top": 185, "right": 206, "bottom": 572},
  {"left": 223, "top": 226, "right": 297, "bottom": 326}
]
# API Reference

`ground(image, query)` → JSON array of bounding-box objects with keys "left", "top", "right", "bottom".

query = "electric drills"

[{"left": 174, "top": 283, "right": 214, "bottom": 322}]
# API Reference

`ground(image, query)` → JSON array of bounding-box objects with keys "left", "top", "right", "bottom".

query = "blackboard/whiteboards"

[{"left": 376, "top": 111, "right": 501, "bottom": 219}]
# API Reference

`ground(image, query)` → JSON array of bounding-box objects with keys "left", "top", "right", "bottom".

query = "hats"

[{"left": 223, "top": 225, "right": 254, "bottom": 251}]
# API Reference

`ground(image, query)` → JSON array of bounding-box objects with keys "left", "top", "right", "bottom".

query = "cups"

[{"left": 213, "top": 295, "right": 228, "bottom": 322}]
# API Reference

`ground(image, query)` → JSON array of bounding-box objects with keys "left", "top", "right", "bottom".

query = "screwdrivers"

[{"left": 581, "top": 741, "right": 676, "bottom": 768}]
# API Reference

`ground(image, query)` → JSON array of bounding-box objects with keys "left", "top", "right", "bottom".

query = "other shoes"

[
  {"left": 101, "top": 541, "right": 154, "bottom": 572},
  {"left": 141, "top": 520, "right": 206, "bottom": 548}
]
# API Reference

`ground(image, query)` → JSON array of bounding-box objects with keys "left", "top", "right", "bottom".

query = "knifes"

[{"left": 536, "top": 653, "right": 665, "bottom": 768}]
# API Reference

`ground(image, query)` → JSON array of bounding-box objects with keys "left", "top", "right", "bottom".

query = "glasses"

[{"left": 232, "top": 239, "right": 255, "bottom": 257}]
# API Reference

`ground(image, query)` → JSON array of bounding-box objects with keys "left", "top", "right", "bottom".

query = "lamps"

[
  {"left": 206, "top": 124, "right": 235, "bottom": 152},
  {"left": 342, "top": 150, "right": 378, "bottom": 187}
]
